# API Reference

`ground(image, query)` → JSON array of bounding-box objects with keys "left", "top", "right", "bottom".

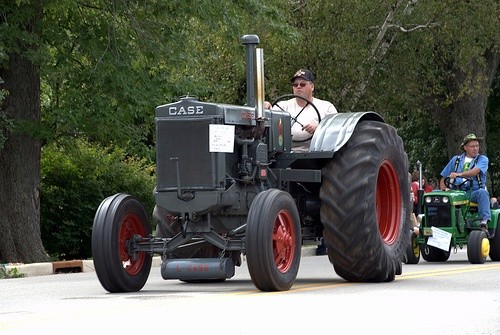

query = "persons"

[
  {"left": 265, "top": 69, "right": 338, "bottom": 152},
  {"left": 440, "top": 133, "right": 492, "bottom": 230}
]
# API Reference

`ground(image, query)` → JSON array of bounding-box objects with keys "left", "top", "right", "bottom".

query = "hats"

[
  {"left": 290, "top": 69, "right": 315, "bottom": 82},
  {"left": 460, "top": 133, "right": 485, "bottom": 148}
]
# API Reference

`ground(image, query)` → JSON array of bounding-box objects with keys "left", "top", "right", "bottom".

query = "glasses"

[
  {"left": 291, "top": 82, "right": 313, "bottom": 87},
  {"left": 429, "top": 180, "right": 433, "bottom": 182}
]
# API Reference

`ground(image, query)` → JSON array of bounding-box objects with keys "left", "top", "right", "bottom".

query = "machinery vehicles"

[
  {"left": 404, "top": 158, "right": 500, "bottom": 264},
  {"left": 90, "top": 33, "right": 416, "bottom": 292}
]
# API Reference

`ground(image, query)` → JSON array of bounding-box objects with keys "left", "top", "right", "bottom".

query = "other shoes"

[{"left": 481, "top": 222, "right": 488, "bottom": 229}]
